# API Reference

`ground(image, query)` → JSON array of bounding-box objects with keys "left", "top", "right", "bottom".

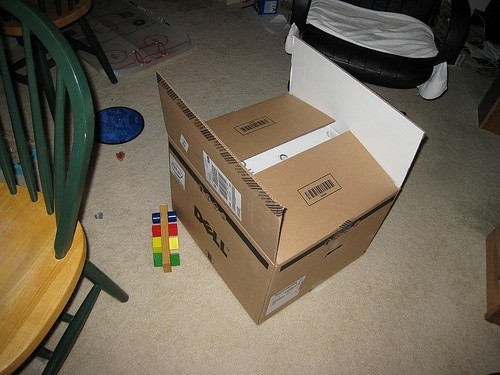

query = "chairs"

[
  {"left": 0, "top": 0, "right": 130, "bottom": 375},
  {"left": 0, "top": 0, "right": 119, "bottom": 120},
  {"left": 287, "top": 0, "right": 471, "bottom": 92}
]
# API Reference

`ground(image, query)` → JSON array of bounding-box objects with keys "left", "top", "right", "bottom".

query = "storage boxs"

[
  {"left": 477, "top": 80, "right": 500, "bottom": 134},
  {"left": 155, "top": 36, "right": 426, "bottom": 326},
  {"left": 254, "top": 0, "right": 279, "bottom": 15}
]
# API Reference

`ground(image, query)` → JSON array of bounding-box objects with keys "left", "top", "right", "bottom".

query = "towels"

[{"left": 284, "top": 0, "right": 449, "bottom": 101}]
